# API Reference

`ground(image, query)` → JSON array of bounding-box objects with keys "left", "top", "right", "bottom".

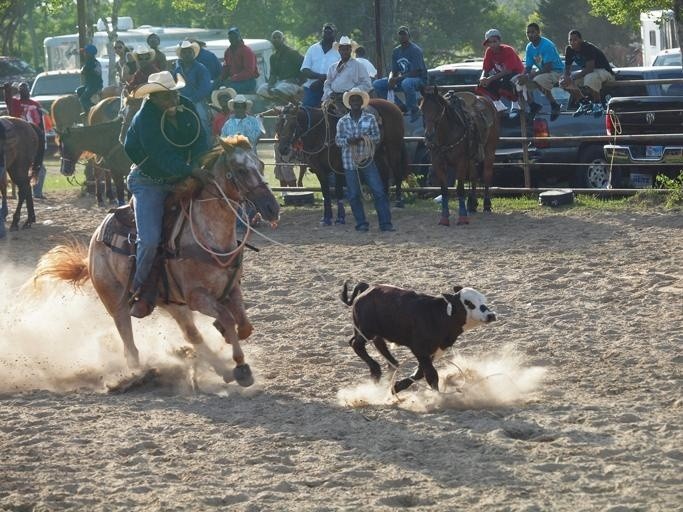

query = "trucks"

[{"left": 44, "top": 17, "right": 271, "bottom": 100}]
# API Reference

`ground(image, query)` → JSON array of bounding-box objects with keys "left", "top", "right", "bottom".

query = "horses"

[
  {"left": 53, "top": 116, "right": 137, "bottom": 210},
  {"left": 272, "top": 99, "right": 404, "bottom": 227},
  {"left": 87, "top": 96, "right": 121, "bottom": 204},
  {"left": 0, "top": 116, "right": 45, "bottom": 232},
  {"left": 418, "top": 84, "right": 498, "bottom": 226},
  {"left": 50, "top": 85, "right": 121, "bottom": 148},
  {"left": 33, "top": 132, "right": 279, "bottom": 387}
]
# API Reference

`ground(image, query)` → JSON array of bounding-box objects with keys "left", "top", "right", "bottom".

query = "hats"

[
  {"left": 399, "top": 26, "right": 409, "bottom": 34},
  {"left": 80, "top": 44, "right": 97, "bottom": 55},
  {"left": 333, "top": 36, "right": 359, "bottom": 50},
  {"left": 129, "top": 70, "right": 186, "bottom": 99},
  {"left": 133, "top": 45, "right": 156, "bottom": 63},
  {"left": 482, "top": 29, "right": 501, "bottom": 45},
  {"left": 211, "top": 86, "right": 253, "bottom": 113},
  {"left": 176, "top": 41, "right": 201, "bottom": 60},
  {"left": 342, "top": 88, "right": 370, "bottom": 110}
]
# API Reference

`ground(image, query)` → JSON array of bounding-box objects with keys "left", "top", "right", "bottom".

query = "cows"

[{"left": 340, "top": 278, "right": 497, "bottom": 396}]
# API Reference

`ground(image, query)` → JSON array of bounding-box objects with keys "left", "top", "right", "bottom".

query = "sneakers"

[
  {"left": 409, "top": 110, "right": 424, "bottom": 123},
  {"left": 382, "top": 224, "right": 394, "bottom": 231},
  {"left": 359, "top": 224, "right": 367, "bottom": 230},
  {"left": 586, "top": 104, "right": 605, "bottom": 115},
  {"left": 550, "top": 103, "right": 561, "bottom": 122},
  {"left": 128, "top": 297, "right": 155, "bottom": 319},
  {"left": 573, "top": 100, "right": 593, "bottom": 118},
  {"left": 498, "top": 105, "right": 522, "bottom": 121},
  {"left": 528, "top": 103, "right": 542, "bottom": 122}
]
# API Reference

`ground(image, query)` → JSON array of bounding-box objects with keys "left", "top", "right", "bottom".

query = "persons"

[
  {"left": 559, "top": 29, "right": 615, "bottom": 118},
  {"left": 516, "top": 23, "right": 564, "bottom": 121},
  {"left": 124, "top": 71, "right": 209, "bottom": 318},
  {"left": 477, "top": 28, "right": 525, "bottom": 119},
  {"left": 4, "top": 23, "right": 429, "bottom": 232}
]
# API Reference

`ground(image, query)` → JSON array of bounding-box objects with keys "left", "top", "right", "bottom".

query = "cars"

[{"left": 1, "top": 56, "right": 99, "bottom": 154}]
